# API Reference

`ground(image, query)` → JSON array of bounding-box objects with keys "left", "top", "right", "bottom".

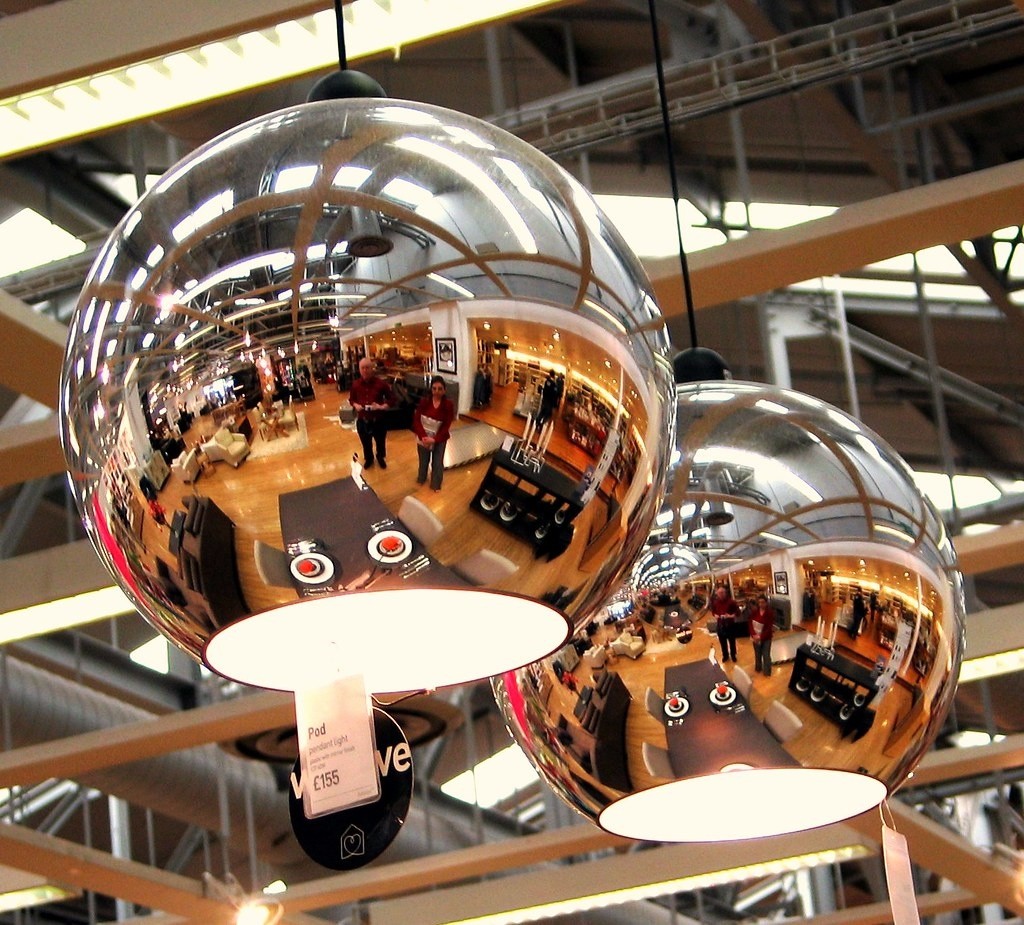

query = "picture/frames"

[
  {"left": 774, "top": 572, "right": 789, "bottom": 595},
  {"left": 434, "top": 338, "right": 458, "bottom": 374}
]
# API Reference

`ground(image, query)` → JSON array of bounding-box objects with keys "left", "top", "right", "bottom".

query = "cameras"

[
  {"left": 720, "top": 615, "right": 725, "bottom": 619},
  {"left": 364, "top": 405, "right": 373, "bottom": 411}
]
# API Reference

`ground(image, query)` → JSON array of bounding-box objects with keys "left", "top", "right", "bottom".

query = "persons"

[
  {"left": 748, "top": 595, "right": 776, "bottom": 679},
  {"left": 870, "top": 589, "right": 877, "bottom": 622},
  {"left": 414, "top": 375, "right": 455, "bottom": 491},
  {"left": 348, "top": 358, "right": 392, "bottom": 469},
  {"left": 555, "top": 373, "right": 563, "bottom": 410},
  {"left": 849, "top": 586, "right": 865, "bottom": 640},
  {"left": 535, "top": 369, "right": 556, "bottom": 429},
  {"left": 711, "top": 587, "right": 741, "bottom": 662}
]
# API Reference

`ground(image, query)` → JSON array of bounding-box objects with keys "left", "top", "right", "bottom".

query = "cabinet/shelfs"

[
  {"left": 470, "top": 441, "right": 586, "bottom": 560},
  {"left": 787, "top": 641, "right": 879, "bottom": 738}
]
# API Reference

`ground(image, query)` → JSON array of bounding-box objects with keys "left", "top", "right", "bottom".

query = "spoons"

[
  {"left": 401, "top": 554, "right": 425, "bottom": 570},
  {"left": 370, "top": 518, "right": 393, "bottom": 532}
]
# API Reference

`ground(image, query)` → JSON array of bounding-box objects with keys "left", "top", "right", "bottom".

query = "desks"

[
  {"left": 261, "top": 402, "right": 290, "bottom": 442},
  {"left": 663, "top": 658, "right": 799, "bottom": 780},
  {"left": 663, "top": 604, "right": 689, "bottom": 628},
  {"left": 279, "top": 476, "right": 469, "bottom": 601}
]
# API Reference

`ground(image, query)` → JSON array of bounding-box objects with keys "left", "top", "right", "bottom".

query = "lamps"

[
  {"left": 810, "top": 616, "right": 839, "bottom": 661},
  {"left": 486, "top": 0, "right": 964, "bottom": 841},
  {"left": 631, "top": 521, "right": 716, "bottom": 630},
  {"left": 62, "top": 0, "right": 676, "bottom": 703},
  {"left": 511, "top": 412, "right": 557, "bottom": 472}
]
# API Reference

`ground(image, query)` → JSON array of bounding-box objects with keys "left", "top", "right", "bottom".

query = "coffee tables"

[
  {"left": 168, "top": 510, "right": 187, "bottom": 555},
  {"left": 231, "top": 415, "right": 253, "bottom": 442},
  {"left": 631, "top": 619, "right": 646, "bottom": 640}
]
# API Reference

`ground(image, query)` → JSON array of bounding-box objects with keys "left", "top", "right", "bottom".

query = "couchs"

[
  {"left": 583, "top": 615, "right": 647, "bottom": 669},
  {"left": 558, "top": 670, "right": 631, "bottom": 794},
  {"left": 155, "top": 494, "right": 251, "bottom": 633},
  {"left": 171, "top": 415, "right": 251, "bottom": 484}
]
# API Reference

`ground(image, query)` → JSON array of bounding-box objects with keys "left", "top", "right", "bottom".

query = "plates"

[
  {"left": 720, "top": 763, "right": 755, "bottom": 772},
  {"left": 710, "top": 686, "right": 737, "bottom": 706},
  {"left": 665, "top": 698, "right": 690, "bottom": 718},
  {"left": 290, "top": 553, "right": 335, "bottom": 584},
  {"left": 367, "top": 529, "right": 413, "bottom": 563}
]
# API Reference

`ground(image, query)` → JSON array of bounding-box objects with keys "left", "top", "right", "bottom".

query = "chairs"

[
  {"left": 454, "top": 550, "right": 518, "bottom": 589},
  {"left": 254, "top": 539, "right": 293, "bottom": 590},
  {"left": 730, "top": 664, "right": 754, "bottom": 705},
  {"left": 252, "top": 395, "right": 299, "bottom": 442},
  {"left": 641, "top": 742, "right": 676, "bottom": 779},
  {"left": 759, "top": 699, "right": 802, "bottom": 744},
  {"left": 645, "top": 686, "right": 663, "bottom": 723},
  {"left": 396, "top": 494, "right": 443, "bottom": 550}
]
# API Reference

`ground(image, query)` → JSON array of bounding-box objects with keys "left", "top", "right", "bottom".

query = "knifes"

[{"left": 398, "top": 558, "right": 429, "bottom": 579}]
